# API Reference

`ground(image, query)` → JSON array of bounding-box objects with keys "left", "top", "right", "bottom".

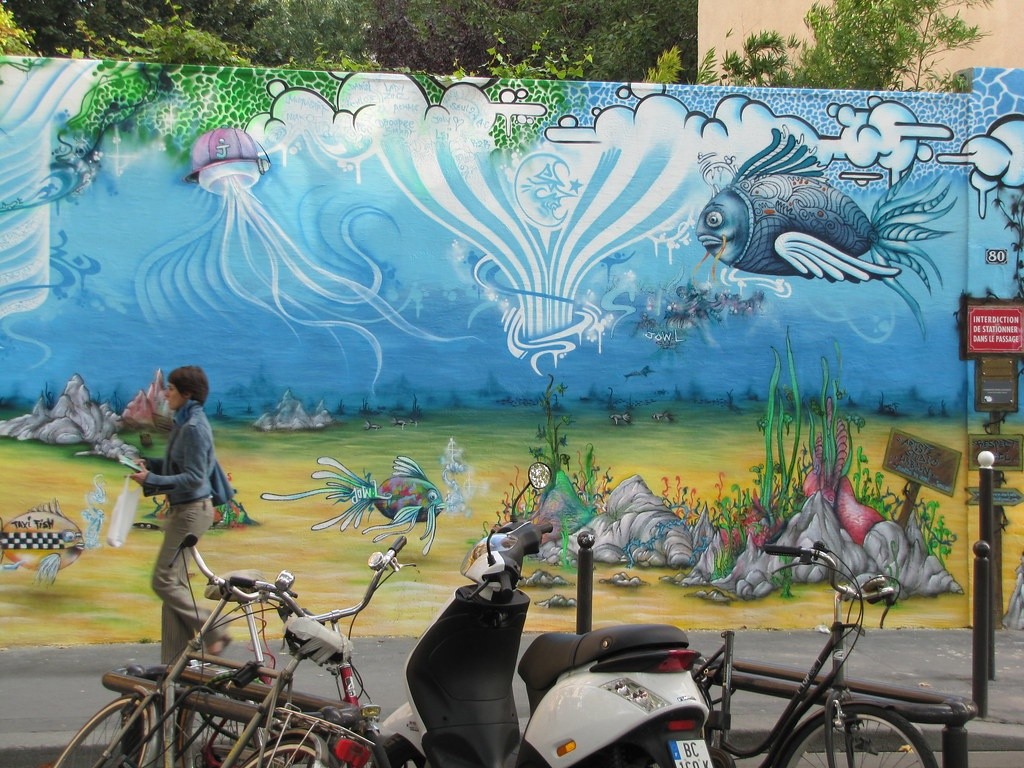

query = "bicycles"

[
  {"left": 50, "top": 534, "right": 417, "bottom": 768},
  {"left": 694, "top": 543, "right": 939, "bottom": 768}
]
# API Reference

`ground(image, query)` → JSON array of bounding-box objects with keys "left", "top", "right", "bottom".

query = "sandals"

[{"left": 207, "top": 634, "right": 234, "bottom": 667}]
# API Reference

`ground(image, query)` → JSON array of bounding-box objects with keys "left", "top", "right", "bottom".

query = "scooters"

[{"left": 380, "top": 462, "right": 714, "bottom": 768}]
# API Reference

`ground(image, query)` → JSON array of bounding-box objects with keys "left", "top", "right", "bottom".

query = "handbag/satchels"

[{"left": 105, "top": 474, "right": 145, "bottom": 549}]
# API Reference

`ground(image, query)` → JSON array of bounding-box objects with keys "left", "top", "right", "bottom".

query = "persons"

[{"left": 130, "top": 365, "right": 232, "bottom": 666}]
